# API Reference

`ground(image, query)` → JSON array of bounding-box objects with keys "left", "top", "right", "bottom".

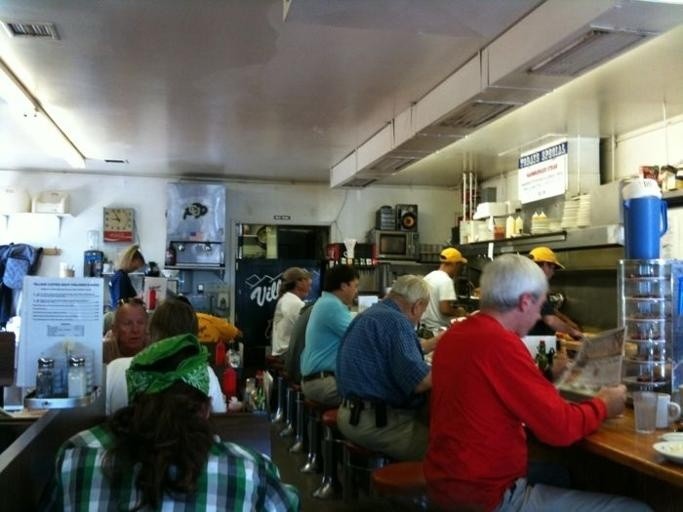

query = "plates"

[
  {"left": 652, "top": 441, "right": 682, "bottom": 463},
  {"left": 529, "top": 218, "right": 557, "bottom": 233},
  {"left": 660, "top": 432, "right": 682, "bottom": 440},
  {"left": 560, "top": 194, "right": 590, "bottom": 229}
]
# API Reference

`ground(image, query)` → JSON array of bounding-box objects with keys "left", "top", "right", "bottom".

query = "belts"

[{"left": 304, "top": 371, "right": 333, "bottom": 381}]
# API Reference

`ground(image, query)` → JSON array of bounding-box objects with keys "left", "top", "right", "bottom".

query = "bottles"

[
  {"left": 67, "top": 357, "right": 85, "bottom": 397},
  {"left": 503, "top": 214, "right": 523, "bottom": 239},
  {"left": 34, "top": 357, "right": 55, "bottom": 396},
  {"left": 547, "top": 348, "right": 556, "bottom": 367},
  {"left": 532, "top": 210, "right": 546, "bottom": 218},
  {"left": 535, "top": 339, "right": 548, "bottom": 372}
]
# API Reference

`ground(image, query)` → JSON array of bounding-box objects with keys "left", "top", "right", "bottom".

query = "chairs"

[{"left": 211, "top": 409, "right": 271, "bottom": 458}]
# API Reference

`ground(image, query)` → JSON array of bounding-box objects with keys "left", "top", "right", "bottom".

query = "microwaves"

[{"left": 366, "top": 228, "right": 421, "bottom": 260}]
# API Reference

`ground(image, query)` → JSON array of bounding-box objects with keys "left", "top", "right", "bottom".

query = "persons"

[
  {"left": 262, "top": 267, "right": 360, "bottom": 409},
  {"left": 424, "top": 253, "right": 652, "bottom": 510},
  {"left": 108, "top": 243, "right": 144, "bottom": 311},
  {"left": 419, "top": 247, "right": 468, "bottom": 336},
  {"left": 43, "top": 333, "right": 301, "bottom": 510},
  {"left": 105, "top": 296, "right": 230, "bottom": 416},
  {"left": 527, "top": 247, "right": 584, "bottom": 340},
  {"left": 103, "top": 300, "right": 150, "bottom": 365},
  {"left": 333, "top": 274, "right": 445, "bottom": 509}
]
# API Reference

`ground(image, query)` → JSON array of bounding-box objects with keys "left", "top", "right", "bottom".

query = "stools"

[{"left": 259, "top": 369, "right": 434, "bottom": 511}]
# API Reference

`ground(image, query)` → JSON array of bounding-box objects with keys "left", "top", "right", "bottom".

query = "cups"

[
  {"left": 631, "top": 390, "right": 660, "bottom": 432},
  {"left": 342, "top": 239, "right": 358, "bottom": 259},
  {"left": 655, "top": 392, "right": 681, "bottom": 429},
  {"left": 622, "top": 196, "right": 669, "bottom": 258}
]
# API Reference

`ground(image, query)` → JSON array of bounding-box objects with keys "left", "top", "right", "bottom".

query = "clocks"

[{"left": 101, "top": 206, "right": 136, "bottom": 244}]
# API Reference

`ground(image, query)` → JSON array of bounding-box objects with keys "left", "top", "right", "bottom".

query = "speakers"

[{"left": 396, "top": 204, "right": 418, "bottom": 232}]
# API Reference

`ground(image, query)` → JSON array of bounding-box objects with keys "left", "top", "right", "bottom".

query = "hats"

[
  {"left": 282, "top": 267, "right": 314, "bottom": 285},
  {"left": 529, "top": 246, "right": 566, "bottom": 271},
  {"left": 439, "top": 247, "right": 468, "bottom": 264}
]
